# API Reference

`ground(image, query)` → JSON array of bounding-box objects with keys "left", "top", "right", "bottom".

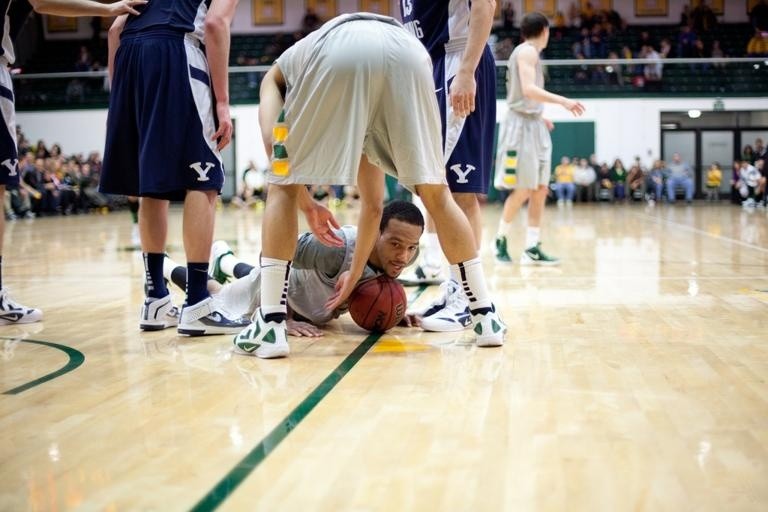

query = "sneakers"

[
  {"left": 410, "top": 280, "right": 458, "bottom": 319},
  {"left": 393, "top": 259, "right": 448, "bottom": 288},
  {"left": 163, "top": 253, "right": 172, "bottom": 288},
  {"left": 177, "top": 297, "right": 251, "bottom": 336},
  {"left": 492, "top": 234, "right": 513, "bottom": 265},
  {"left": 419, "top": 295, "right": 472, "bottom": 332},
  {"left": 139, "top": 295, "right": 180, "bottom": 332},
  {"left": 230, "top": 305, "right": 289, "bottom": 359},
  {"left": 520, "top": 241, "right": 560, "bottom": 266},
  {"left": 208, "top": 240, "right": 234, "bottom": 286},
  {"left": 470, "top": 312, "right": 508, "bottom": 347},
  {"left": 0, "top": 289, "right": 45, "bottom": 328}
]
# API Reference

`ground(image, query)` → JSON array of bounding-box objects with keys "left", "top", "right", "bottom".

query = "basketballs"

[{"left": 349, "top": 277, "right": 406, "bottom": 332}]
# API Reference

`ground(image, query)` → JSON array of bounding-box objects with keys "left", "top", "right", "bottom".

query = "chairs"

[
  {"left": 226, "top": 34, "right": 298, "bottom": 105},
  {"left": 543, "top": 35, "right": 767, "bottom": 97},
  {"left": 550, "top": 169, "right": 687, "bottom": 201},
  {"left": 495, "top": 66, "right": 507, "bottom": 101},
  {"left": 13, "top": 37, "right": 107, "bottom": 111}
]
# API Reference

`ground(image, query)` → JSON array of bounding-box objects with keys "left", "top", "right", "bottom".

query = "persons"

[
  {"left": 143, "top": 200, "right": 425, "bottom": 336},
  {"left": 0, "top": 1, "right": 150, "bottom": 324},
  {"left": 97, "top": 1, "right": 246, "bottom": 334},
  {"left": 230, "top": 11, "right": 507, "bottom": 358},
  {"left": 398, "top": 0, "right": 499, "bottom": 335}
]
